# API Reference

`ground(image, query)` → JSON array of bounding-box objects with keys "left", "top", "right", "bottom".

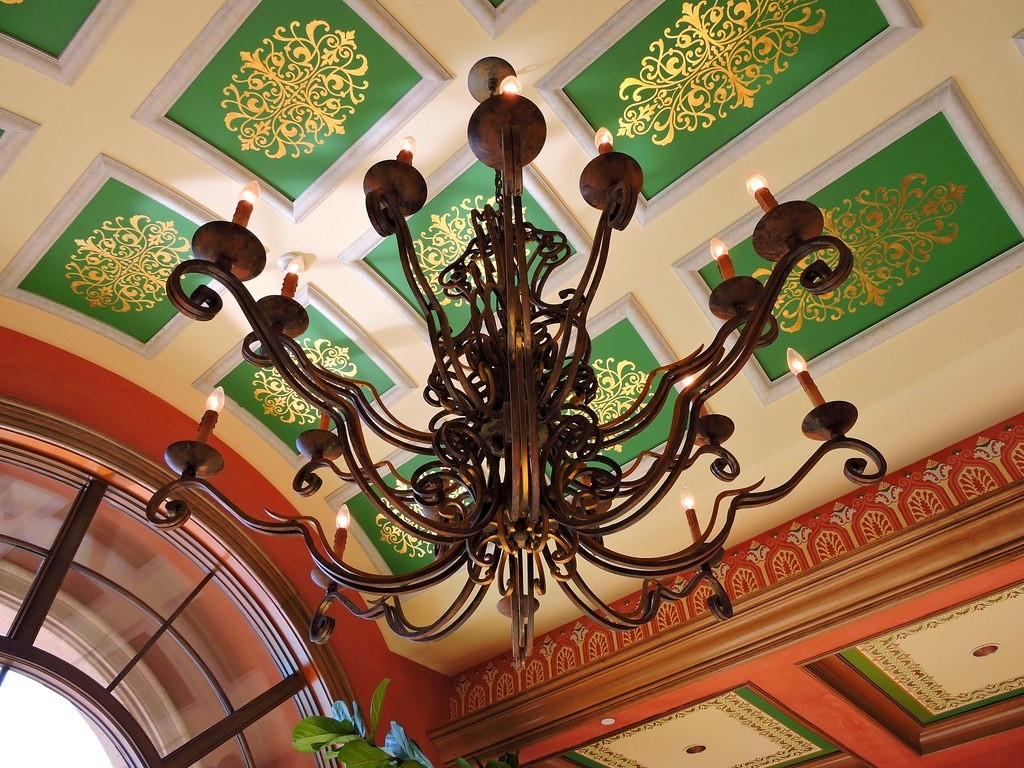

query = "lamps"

[{"left": 141, "top": 54, "right": 888, "bottom": 660}]
